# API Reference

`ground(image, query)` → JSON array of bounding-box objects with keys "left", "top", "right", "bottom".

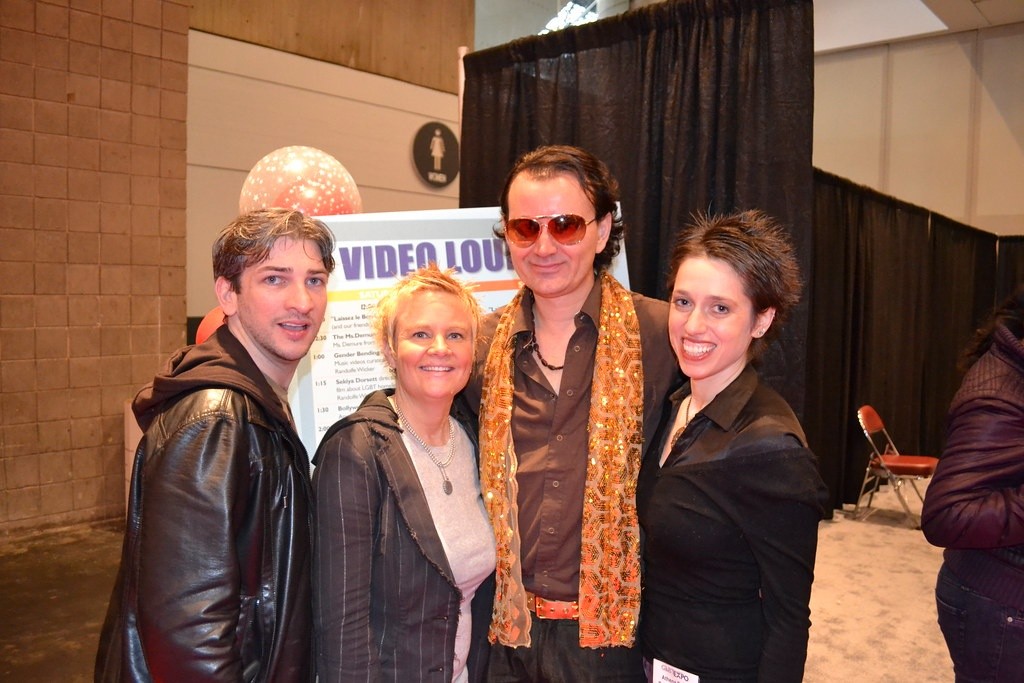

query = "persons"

[
  {"left": 311, "top": 258, "right": 496, "bottom": 683},
  {"left": 636, "top": 209, "right": 820, "bottom": 683},
  {"left": 93, "top": 208, "right": 335, "bottom": 683},
  {"left": 449, "top": 145, "right": 691, "bottom": 683},
  {"left": 920, "top": 282, "right": 1024, "bottom": 683}
]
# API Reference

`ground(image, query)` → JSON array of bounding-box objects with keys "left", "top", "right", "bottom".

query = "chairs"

[{"left": 856, "top": 404, "right": 939, "bottom": 531}]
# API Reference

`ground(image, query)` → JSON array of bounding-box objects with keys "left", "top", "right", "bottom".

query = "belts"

[{"left": 526, "top": 592, "right": 579, "bottom": 620}]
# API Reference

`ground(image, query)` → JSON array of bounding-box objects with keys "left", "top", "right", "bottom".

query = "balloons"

[
  {"left": 195, "top": 305, "right": 224, "bottom": 347},
  {"left": 237, "top": 144, "right": 362, "bottom": 217}
]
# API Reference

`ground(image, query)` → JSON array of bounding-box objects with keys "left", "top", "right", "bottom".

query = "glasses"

[{"left": 502, "top": 212, "right": 606, "bottom": 249}]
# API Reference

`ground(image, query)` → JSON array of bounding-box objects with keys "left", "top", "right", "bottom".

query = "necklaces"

[
  {"left": 671, "top": 397, "right": 692, "bottom": 450},
  {"left": 395, "top": 396, "right": 455, "bottom": 495},
  {"left": 531, "top": 293, "right": 564, "bottom": 370}
]
